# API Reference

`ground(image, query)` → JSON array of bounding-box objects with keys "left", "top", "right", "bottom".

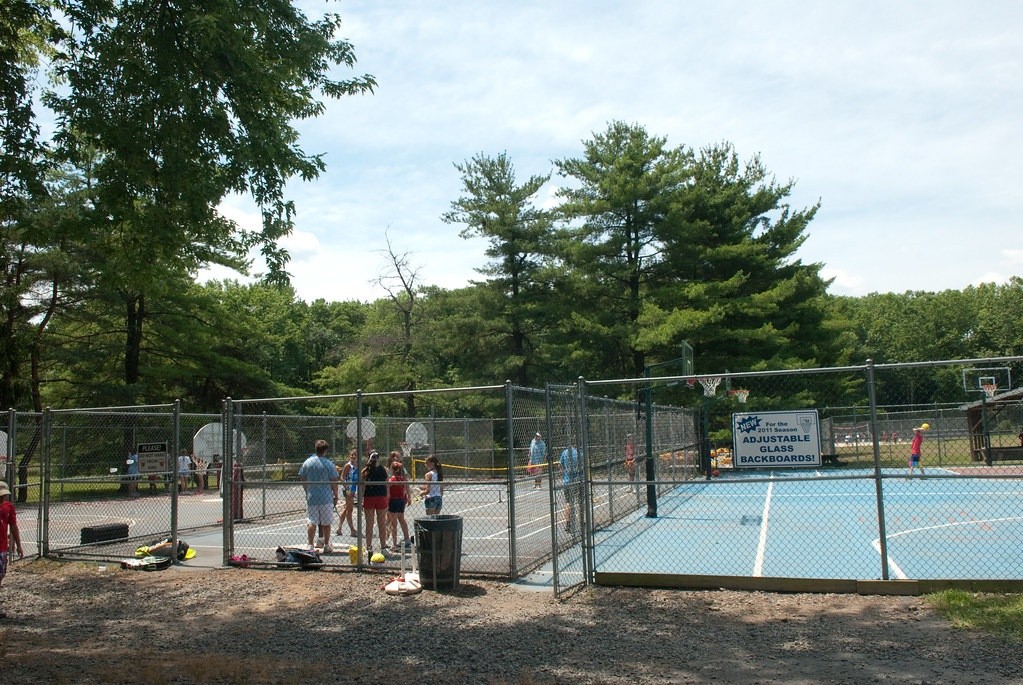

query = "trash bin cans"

[{"left": 412, "top": 514, "right": 463, "bottom": 592}]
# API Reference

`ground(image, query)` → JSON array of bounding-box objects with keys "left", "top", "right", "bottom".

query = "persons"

[
  {"left": 0, "top": 481, "right": 23, "bottom": 582},
  {"left": 560, "top": 435, "right": 586, "bottom": 531},
  {"left": 627, "top": 434, "right": 639, "bottom": 493},
  {"left": 419, "top": 455, "right": 443, "bottom": 514},
  {"left": 389, "top": 462, "right": 411, "bottom": 550},
  {"left": 834, "top": 431, "right": 897, "bottom": 446},
  {"left": 1019, "top": 428, "right": 1023, "bottom": 446},
  {"left": 385, "top": 451, "right": 408, "bottom": 539},
  {"left": 178, "top": 449, "right": 209, "bottom": 495},
  {"left": 129, "top": 451, "right": 173, "bottom": 493},
  {"left": 910, "top": 427, "right": 926, "bottom": 474},
  {"left": 528, "top": 433, "right": 547, "bottom": 490},
  {"left": 298, "top": 440, "right": 338, "bottom": 553},
  {"left": 336, "top": 449, "right": 363, "bottom": 537},
  {"left": 361, "top": 449, "right": 389, "bottom": 550}
]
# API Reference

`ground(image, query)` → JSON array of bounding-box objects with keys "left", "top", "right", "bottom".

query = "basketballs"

[{"left": 371, "top": 553, "right": 385, "bottom": 562}]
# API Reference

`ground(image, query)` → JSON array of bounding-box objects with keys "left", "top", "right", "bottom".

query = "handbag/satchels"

[
  {"left": 135, "top": 535, "right": 187, "bottom": 559},
  {"left": 276, "top": 544, "right": 324, "bottom": 571}
]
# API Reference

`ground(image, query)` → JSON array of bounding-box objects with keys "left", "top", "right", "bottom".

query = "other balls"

[{"left": 921, "top": 425, "right": 929, "bottom": 432}]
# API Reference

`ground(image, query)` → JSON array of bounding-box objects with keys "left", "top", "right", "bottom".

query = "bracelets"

[{"left": 346, "top": 490, "right": 350, "bottom": 494}]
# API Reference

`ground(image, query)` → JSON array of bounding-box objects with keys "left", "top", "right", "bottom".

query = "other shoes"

[
  {"left": 398, "top": 539, "right": 411, "bottom": 547},
  {"left": 180, "top": 491, "right": 189, "bottom": 495},
  {"left": 390, "top": 544, "right": 399, "bottom": 552},
  {"left": 350, "top": 530, "right": 362, "bottom": 537},
  {"left": 325, "top": 546, "right": 333, "bottom": 555},
  {"left": 335, "top": 529, "right": 342, "bottom": 535}
]
